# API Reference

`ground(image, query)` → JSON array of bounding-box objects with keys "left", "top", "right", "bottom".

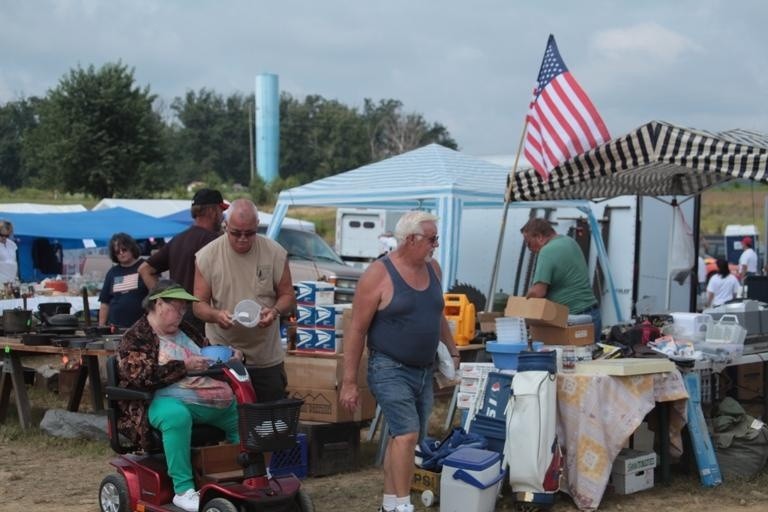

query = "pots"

[{"left": 3, "top": 307, "right": 32, "bottom": 335}]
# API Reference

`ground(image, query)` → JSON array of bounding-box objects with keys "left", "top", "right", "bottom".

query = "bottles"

[
  {"left": 642, "top": 320, "right": 651, "bottom": 345},
  {"left": 55, "top": 273, "right": 62, "bottom": 281},
  {"left": 561, "top": 347, "right": 576, "bottom": 372},
  {"left": 69, "top": 270, "right": 106, "bottom": 295},
  {"left": 0, "top": 277, "right": 35, "bottom": 299}
]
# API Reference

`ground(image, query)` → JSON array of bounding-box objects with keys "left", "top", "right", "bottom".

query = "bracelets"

[
  {"left": 273, "top": 307, "right": 280, "bottom": 316},
  {"left": 451, "top": 354, "right": 460, "bottom": 357}
]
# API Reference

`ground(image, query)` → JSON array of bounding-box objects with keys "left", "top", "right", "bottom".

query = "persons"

[
  {"left": 192, "top": 199, "right": 296, "bottom": 404},
  {"left": 338, "top": 210, "right": 461, "bottom": 512},
  {"left": 696, "top": 237, "right": 757, "bottom": 312},
  {"left": 117, "top": 278, "right": 245, "bottom": 512},
  {"left": 520, "top": 218, "right": 603, "bottom": 338},
  {"left": 136, "top": 187, "right": 230, "bottom": 342},
  {"left": 0, "top": 220, "right": 19, "bottom": 301},
  {"left": 97, "top": 233, "right": 162, "bottom": 336}
]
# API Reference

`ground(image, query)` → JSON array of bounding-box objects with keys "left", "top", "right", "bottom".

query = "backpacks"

[{"left": 31, "top": 238, "right": 62, "bottom": 274}]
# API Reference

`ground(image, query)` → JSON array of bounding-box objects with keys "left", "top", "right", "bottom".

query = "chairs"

[{"left": 105, "top": 356, "right": 230, "bottom": 452}]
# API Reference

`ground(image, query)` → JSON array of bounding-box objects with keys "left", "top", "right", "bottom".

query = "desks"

[
  {"left": 475, "top": 371, "right": 677, "bottom": 487},
  {"left": 644, "top": 337, "right": 767, "bottom": 471},
  {"left": 0, "top": 295, "right": 98, "bottom": 318},
  {"left": 1, "top": 337, "right": 107, "bottom": 435}
]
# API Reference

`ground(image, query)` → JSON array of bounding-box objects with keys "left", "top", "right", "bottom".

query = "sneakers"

[{"left": 173, "top": 489, "right": 202, "bottom": 511}]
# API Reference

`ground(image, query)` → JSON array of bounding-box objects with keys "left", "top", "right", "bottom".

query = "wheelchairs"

[{"left": 96, "top": 343, "right": 317, "bottom": 512}]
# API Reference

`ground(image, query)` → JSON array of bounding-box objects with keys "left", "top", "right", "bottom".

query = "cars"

[{"left": 698, "top": 251, "right": 742, "bottom": 285}]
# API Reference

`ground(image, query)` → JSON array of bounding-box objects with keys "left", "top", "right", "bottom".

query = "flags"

[
  {"left": 523, "top": 38, "right": 612, "bottom": 180},
  {"left": 113, "top": 272, "right": 140, "bottom": 292}
]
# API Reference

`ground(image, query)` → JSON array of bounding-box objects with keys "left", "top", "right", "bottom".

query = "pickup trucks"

[{"left": 73, "top": 218, "right": 367, "bottom": 305}]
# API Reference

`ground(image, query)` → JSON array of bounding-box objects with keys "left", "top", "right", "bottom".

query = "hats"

[
  {"left": 191, "top": 189, "right": 230, "bottom": 211},
  {"left": 148, "top": 284, "right": 201, "bottom": 303},
  {"left": 740, "top": 237, "right": 753, "bottom": 245}
]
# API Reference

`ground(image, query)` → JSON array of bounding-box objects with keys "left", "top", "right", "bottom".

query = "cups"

[
  {"left": 233, "top": 299, "right": 262, "bottom": 329},
  {"left": 328, "top": 275, "right": 336, "bottom": 286},
  {"left": 531, "top": 341, "right": 544, "bottom": 351}
]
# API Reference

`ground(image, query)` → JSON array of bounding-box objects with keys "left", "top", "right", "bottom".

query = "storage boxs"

[
  {"left": 506, "top": 295, "right": 596, "bottom": 346},
  {"left": 277, "top": 279, "right": 380, "bottom": 475},
  {"left": 475, "top": 310, "right": 505, "bottom": 333},
  {"left": 614, "top": 446, "right": 657, "bottom": 494},
  {"left": 724, "top": 361, "right": 762, "bottom": 400}
]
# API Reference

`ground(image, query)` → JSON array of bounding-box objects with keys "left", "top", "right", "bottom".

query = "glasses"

[
  {"left": 113, "top": 248, "right": 127, "bottom": 255},
  {"left": 412, "top": 233, "right": 439, "bottom": 243},
  {"left": 0, "top": 233, "right": 10, "bottom": 238},
  {"left": 227, "top": 223, "right": 257, "bottom": 238}
]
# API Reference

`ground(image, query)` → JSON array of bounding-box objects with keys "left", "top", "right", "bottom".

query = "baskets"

[
  {"left": 705, "top": 315, "right": 747, "bottom": 345},
  {"left": 647, "top": 342, "right": 720, "bottom": 403},
  {"left": 236, "top": 396, "right": 304, "bottom": 452}
]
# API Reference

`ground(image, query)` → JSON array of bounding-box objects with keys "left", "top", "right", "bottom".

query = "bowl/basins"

[
  {"left": 23, "top": 313, "right": 128, "bottom": 349},
  {"left": 199, "top": 345, "right": 232, "bottom": 362}
]
